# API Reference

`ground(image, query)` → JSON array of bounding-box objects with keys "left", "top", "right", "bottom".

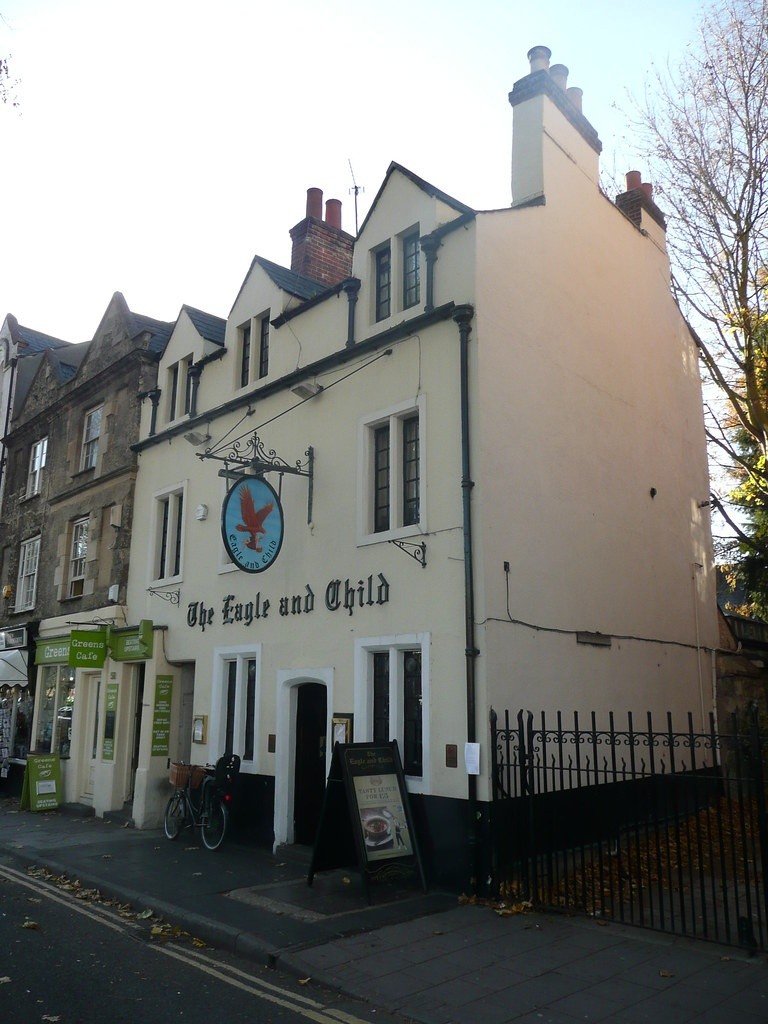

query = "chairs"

[{"left": 201, "top": 754, "right": 241, "bottom": 805}]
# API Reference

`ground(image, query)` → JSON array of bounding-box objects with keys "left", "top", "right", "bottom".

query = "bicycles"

[{"left": 164, "top": 754, "right": 241, "bottom": 851}]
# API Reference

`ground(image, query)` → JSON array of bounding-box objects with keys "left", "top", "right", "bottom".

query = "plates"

[{"left": 365, "top": 830, "right": 392, "bottom": 846}]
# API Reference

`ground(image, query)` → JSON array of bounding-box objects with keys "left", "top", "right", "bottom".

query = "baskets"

[{"left": 169, "top": 763, "right": 204, "bottom": 788}]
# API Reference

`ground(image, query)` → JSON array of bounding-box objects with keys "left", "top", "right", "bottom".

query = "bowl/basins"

[{"left": 363, "top": 816, "right": 390, "bottom": 838}]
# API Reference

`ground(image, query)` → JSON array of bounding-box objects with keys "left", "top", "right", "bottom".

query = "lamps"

[
  {"left": 291, "top": 383, "right": 323, "bottom": 400},
  {"left": 184, "top": 431, "right": 211, "bottom": 446}
]
